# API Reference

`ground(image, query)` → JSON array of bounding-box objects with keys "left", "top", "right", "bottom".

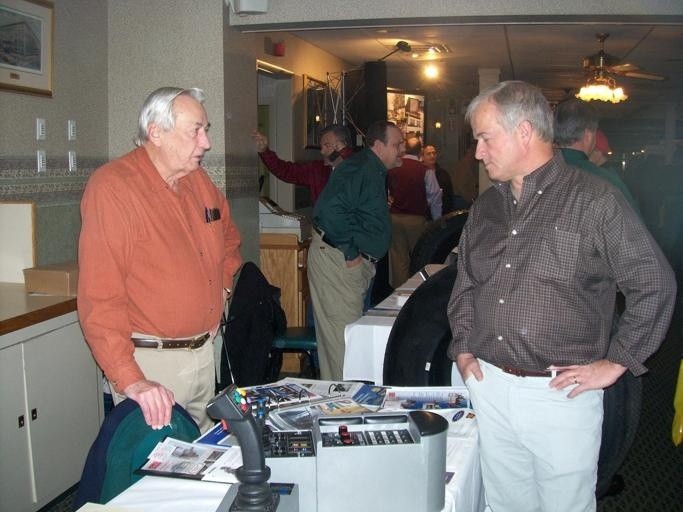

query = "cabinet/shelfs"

[
  {"left": 0, "top": 282, "right": 107, "bottom": 512},
  {"left": 261, "top": 232, "right": 313, "bottom": 375}
]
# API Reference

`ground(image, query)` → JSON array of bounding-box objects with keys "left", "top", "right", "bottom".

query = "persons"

[
  {"left": 254, "top": 123, "right": 375, "bottom": 373},
  {"left": 75, "top": 86, "right": 243, "bottom": 438},
  {"left": 589, "top": 126, "right": 623, "bottom": 181},
  {"left": 386, "top": 136, "right": 443, "bottom": 289},
  {"left": 306, "top": 121, "right": 407, "bottom": 382},
  {"left": 447, "top": 79, "right": 678, "bottom": 512},
  {"left": 552, "top": 96, "right": 642, "bottom": 499},
  {"left": 421, "top": 145, "right": 454, "bottom": 224}
]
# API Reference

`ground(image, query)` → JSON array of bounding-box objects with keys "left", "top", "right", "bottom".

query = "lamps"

[{"left": 572, "top": 49, "right": 630, "bottom": 105}]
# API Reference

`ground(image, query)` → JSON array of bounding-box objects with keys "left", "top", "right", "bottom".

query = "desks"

[
  {"left": 339, "top": 261, "right": 451, "bottom": 384},
  {"left": 74, "top": 376, "right": 487, "bottom": 512}
]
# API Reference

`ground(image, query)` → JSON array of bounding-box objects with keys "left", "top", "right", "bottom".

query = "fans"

[{"left": 531, "top": 32, "right": 670, "bottom": 82}]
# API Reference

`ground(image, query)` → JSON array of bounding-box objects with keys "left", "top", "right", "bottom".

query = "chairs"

[
  {"left": 272, "top": 324, "right": 319, "bottom": 378},
  {"left": 72, "top": 398, "right": 203, "bottom": 512}
]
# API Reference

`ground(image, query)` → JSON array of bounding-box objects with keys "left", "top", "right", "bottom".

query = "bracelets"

[{"left": 224, "top": 286, "right": 231, "bottom": 295}]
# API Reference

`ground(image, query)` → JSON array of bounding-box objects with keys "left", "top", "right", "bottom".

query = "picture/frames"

[
  {"left": 300, "top": 72, "right": 338, "bottom": 152},
  {"left": 0, "top": 0, "right": 57, "bottom": 100}
]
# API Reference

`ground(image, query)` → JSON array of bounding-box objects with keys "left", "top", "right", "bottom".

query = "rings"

[{"left": 572, "top": 377, "right": 577, "bottom": 383}]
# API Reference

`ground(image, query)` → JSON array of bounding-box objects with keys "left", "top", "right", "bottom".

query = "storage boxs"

[{"left": 22, "top": 259, "right": 79, "bottom": 297}]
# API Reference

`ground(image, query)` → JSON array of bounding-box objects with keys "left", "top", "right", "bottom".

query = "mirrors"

[{"left": 385, "top": 89, "right": 429, "bottom": 157}]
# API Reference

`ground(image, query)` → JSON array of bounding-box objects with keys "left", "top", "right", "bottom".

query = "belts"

[
  {"left": 131, "top": 332, "right": 210, "bottom": 350},
  {"left": 503, "top": 365, "right": 559, "bottom": 377},
  {"left": 314, "top": 225, "right": 377, "bottom": 265}
]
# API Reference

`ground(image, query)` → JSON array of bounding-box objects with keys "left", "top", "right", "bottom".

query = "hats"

[{"left": 595, "top": 130, "right": 614, "bottom": 157}]
